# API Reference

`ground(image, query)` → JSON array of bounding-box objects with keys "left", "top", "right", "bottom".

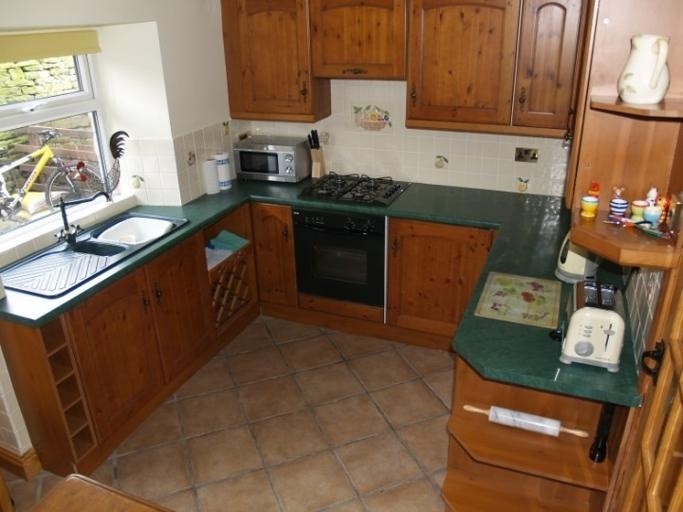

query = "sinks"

[{"left": 90, "top": 212, "right": 189, "bottom": 250}]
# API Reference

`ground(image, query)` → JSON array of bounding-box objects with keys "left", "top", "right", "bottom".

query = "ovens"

[{"left": 292, "top": 206, "right": 387, "bottom": 308}]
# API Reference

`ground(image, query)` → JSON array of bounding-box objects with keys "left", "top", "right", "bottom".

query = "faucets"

[{"left": 54, "top": 191, "right": 112, "bottom": 245}]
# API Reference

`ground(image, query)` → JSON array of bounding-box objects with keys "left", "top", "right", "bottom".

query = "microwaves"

[{"left": 232, "top": 136, "right": 311, "bottom": 183}]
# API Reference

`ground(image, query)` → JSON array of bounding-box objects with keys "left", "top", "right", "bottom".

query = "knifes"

[{"left": 308, "top": 130, "right": 319, "bottom": 149}]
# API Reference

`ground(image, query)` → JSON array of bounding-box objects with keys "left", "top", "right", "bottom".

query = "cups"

[
  {"left": 629, "top": 199, "right": 662, "bottom": 231},
  {"left": 607, "top": 199, "right": 627, "bottom": 224},
  {"left": 580, "top": 195, "right": 598, "bottom": 218}
]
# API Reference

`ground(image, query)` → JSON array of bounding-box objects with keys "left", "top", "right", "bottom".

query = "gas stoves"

[{"left": 298, "top": 172, "right": 411, "bottom": 207}]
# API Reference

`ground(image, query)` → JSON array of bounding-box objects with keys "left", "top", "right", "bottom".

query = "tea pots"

[{"left": 617, "top": 33, "right": 671, "bottom": 105}]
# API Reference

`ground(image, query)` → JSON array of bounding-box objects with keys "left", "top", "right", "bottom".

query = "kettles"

[{"left": 554, "top": 227, "right": 604, "bottom": 284}]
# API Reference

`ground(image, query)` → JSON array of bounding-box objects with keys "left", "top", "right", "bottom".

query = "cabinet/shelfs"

[
  {"left": 219, "top": 0, "right": 330, "bottom": 123},
  {"left": 402, "top": 0, "right": 590, "bottom": 138},
  {"left": 382, "top": 216, "right": 491, "bottom": 347},
  {"left": 251, "top": 195, "right": 299, "bottom": 321},
  {"left": 441, "top": 354, "right": 632, "bottom": 510},
  {"left": 571, "top": 96, "right": 681, "bottom": 273},
  {"left": 308, "top": 1, "right": 407, "bottom": 82}
]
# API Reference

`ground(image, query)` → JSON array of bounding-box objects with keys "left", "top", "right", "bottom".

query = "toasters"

[{"left": 558, "top": 279, "right": 625, "bottom": 373}]
